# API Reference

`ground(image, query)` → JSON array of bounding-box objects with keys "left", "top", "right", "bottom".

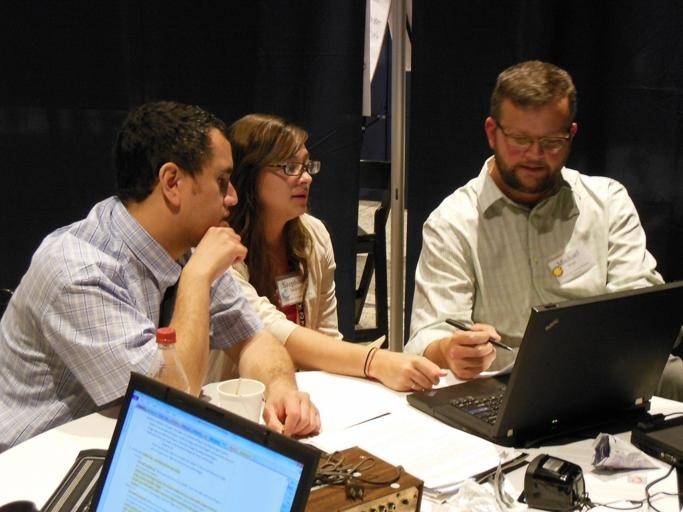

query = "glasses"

[
  {"left": 496, "top": 123, "right": 572, "bottom": 148},
  {"left": 258, "top": 160, "right": 321, "bottom": 176}
]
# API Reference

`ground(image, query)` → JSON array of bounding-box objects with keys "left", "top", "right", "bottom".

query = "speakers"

[{"left": 517, "top": 453, "right": 588, "bottom": 512}]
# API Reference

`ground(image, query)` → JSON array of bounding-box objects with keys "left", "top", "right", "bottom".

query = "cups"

[{"left": 216, "top": 377, "right": 266, "bottom": 421}]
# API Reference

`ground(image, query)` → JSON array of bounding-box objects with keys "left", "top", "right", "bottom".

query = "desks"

[{"left": 0, "top": 369, "right": 681, "bottom": 512}]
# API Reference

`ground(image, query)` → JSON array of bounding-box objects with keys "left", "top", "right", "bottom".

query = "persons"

[
  {"left": 401, "top": 57, "right": 682, "bottom": 408},
  {"left": 199, "top": 112, "right": 445, "bottom": 400},
  {"left": 0, "top": 95, "right": 328, "bottom": 446}
]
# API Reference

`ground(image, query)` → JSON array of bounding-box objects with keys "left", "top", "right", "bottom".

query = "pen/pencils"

[{"left": 445, "top": 319, "right": 513, "bottom": 351}]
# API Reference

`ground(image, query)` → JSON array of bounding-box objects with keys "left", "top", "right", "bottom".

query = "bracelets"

[{"left": 361, "top": 344, "right": 380, "bottom": 380}]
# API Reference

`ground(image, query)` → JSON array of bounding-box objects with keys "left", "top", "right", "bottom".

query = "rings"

[{"left": 410, "top": 382, "right": 416, "bottom": 389}]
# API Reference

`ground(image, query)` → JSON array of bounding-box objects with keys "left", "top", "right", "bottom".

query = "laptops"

[
  {"left": 406, "top": 281, "right": 683, "bottom": 446},
  {"left": 88, "top": 371, "right": 323, "bottom": 512}
]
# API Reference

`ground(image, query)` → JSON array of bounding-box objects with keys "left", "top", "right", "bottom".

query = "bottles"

[{"left": 150, "top": 325, "right": 190, "bottom": 396}]
